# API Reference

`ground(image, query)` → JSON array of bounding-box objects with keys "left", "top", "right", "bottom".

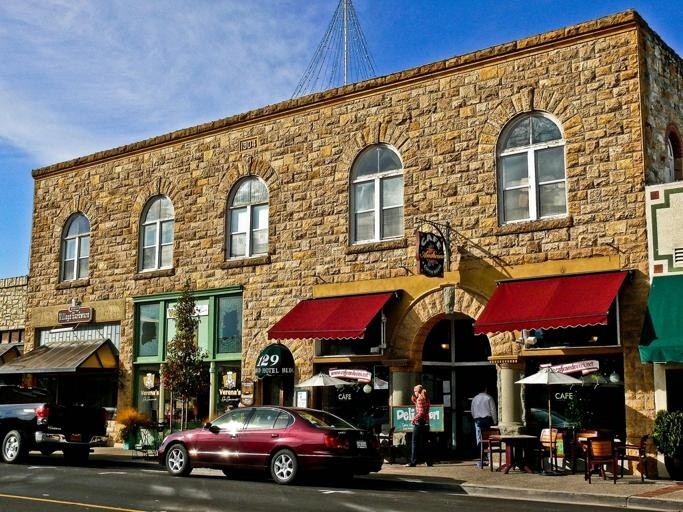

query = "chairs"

[{"left": 477, "top": 426, "right": 648, "bottom": 485}]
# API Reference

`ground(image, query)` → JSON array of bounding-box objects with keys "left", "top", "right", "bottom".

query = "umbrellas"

[
  {"left": 292, "top": 372, "right": 357, "bottom": 412},
  {"left": 512, "top": 365, "right": 583, "bottom": 471}
]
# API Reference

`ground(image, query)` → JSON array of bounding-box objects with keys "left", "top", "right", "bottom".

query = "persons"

[
  {"left": 468, "top": 385, "right": 497, "bottom": 464},
  {"left": 404, "top": 384, "right": 434, "bottom": 467}
]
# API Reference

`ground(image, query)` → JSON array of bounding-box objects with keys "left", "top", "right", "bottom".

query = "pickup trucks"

[{"left": 0, "top": 382, "right": 111, "bottom": 465}]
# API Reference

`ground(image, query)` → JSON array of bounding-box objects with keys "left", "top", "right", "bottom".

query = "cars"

[
  {"left": 529, "top": 405, "right": 581, "bottom": 430},
  {"left": 155, "top": 404, "right": 387, "bottom": 488}
]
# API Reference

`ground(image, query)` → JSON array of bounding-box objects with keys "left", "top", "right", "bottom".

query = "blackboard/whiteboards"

[{"left": 391, "top": 404, "right": 444, "bottom": 432}]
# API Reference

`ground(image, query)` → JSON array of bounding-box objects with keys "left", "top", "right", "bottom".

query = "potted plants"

[{"left": 650, "top": 409, "right": 682, "bottom": 481}]
